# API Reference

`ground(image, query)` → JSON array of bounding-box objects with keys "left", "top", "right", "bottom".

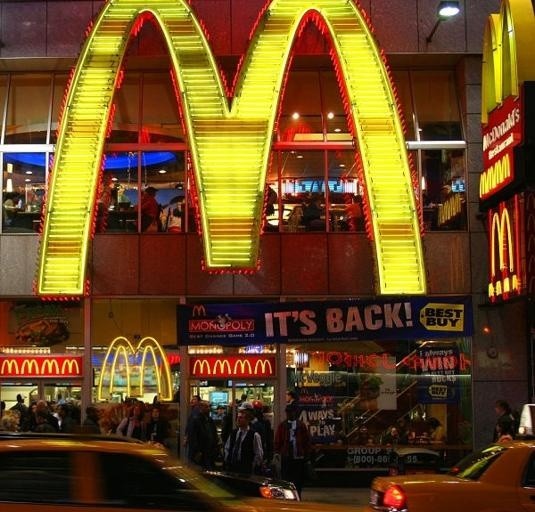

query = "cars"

[
  {"left": 1, "top": 431, "right": 299, "bottom": 503},
  {"left": 370, "top": 432, "right": 535, "bottom": 511},
  {"left": 0, "top": 428, "right": 379, "bottom": 512}
]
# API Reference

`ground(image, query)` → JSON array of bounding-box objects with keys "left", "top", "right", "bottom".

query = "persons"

[
  {"left": 116, "top": 404, "right": 146, "bottom": 441},
  {"left": 232, "top": 394, "right": 272, "bottom": 426},
  {"left": 4, "top": 191, "right": 23, "bottom": 223},
  {"left": 350, "top": 417, "right": 445, "bottom": 447},
  {"left": 493, "top": 400, "right": 514, "bottom": 442},
  {"left": 493, "top": 421, "right": 514, "bottom": 443},
  {"left": 188, "top": 401, "right": 217, "bottom": 469},
  {"left": 166, "top": 196, "right": 184, "bottom": 231},
  {"left": 145, "top": 409, "right": 166, "bottom": 443},
  {"left": 306, "top": 198, "right": 331, "bottom": 228},
  {"left": 136, "top": 186, "right": 168, "bottom": 232},
  {"left": 83, "top": 408, "right": 99, "bottom": 432},
  {"left": 183, "top": 398, "right": 199, "bottom": 447},
  {"left": 340, "top": 196, "right": 364, "bottom": 231},
  {"left": 222, "top": 412, "right": 261, "bottom": 474},
  {"left": 3, "top": 394, "right": 74, "bottom": 433},
  {"left": 275, "top": 405, "right": 310, "bottom": 497}
]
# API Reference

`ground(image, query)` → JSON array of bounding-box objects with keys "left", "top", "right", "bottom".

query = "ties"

[{"left": 228, "top": 429, "right": 244, "bottom": 463}]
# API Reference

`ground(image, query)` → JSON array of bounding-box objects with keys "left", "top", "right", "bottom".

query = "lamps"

[{"left": 427, "top": 1, "right": 459, "bottom": 40}]
